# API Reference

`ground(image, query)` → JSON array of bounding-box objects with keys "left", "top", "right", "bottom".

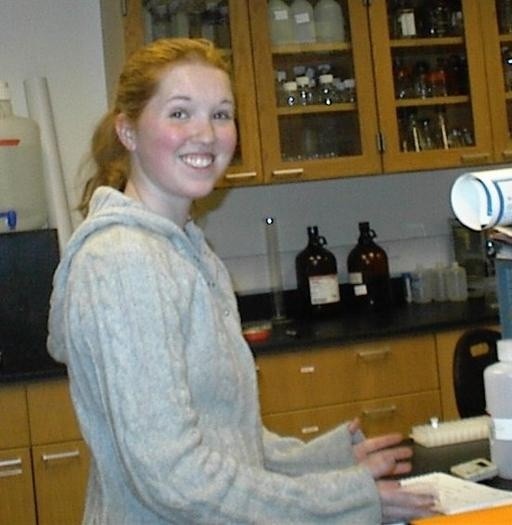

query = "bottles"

[
  {"left": 293, "top": 222, "right": 343, "bottom": 318},
  {"left": 412, "top": 260, "right": 469, "bottom": 305},
  {"left": 274, "top": 62, "right": 358, "bottom": 107},
  {"left": 395, "top": 104, "right": 462, "bottom": 152},
  {"left": 344, "top": 220, "right": 393, "bottom": 311},
  {"left": 393, "top": 53, "right": 469, "bottom": 99},
  {"left": 264, "top": 2, "right": 344, "bottom": 48}
]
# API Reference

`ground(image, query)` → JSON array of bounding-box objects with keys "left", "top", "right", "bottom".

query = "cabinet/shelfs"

[
  {"left": 433, "top": 328, "right": 503, "bottom": 423},
  {"left": 99, "top": 2, "right": 384, "bottom": 188},
  {"left": 366, "top": 2, "right": 511, "bottom": 176},
  {"left": 0, "top": 380, "right": 93, "bottom": 524},
  {"left": 251, "top": 325, "right": 438, "bottom": 455}
]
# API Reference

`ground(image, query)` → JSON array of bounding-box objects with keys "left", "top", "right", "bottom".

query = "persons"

[{"left": 37, "top": 36, "right": 436, "bottom": 525}]
